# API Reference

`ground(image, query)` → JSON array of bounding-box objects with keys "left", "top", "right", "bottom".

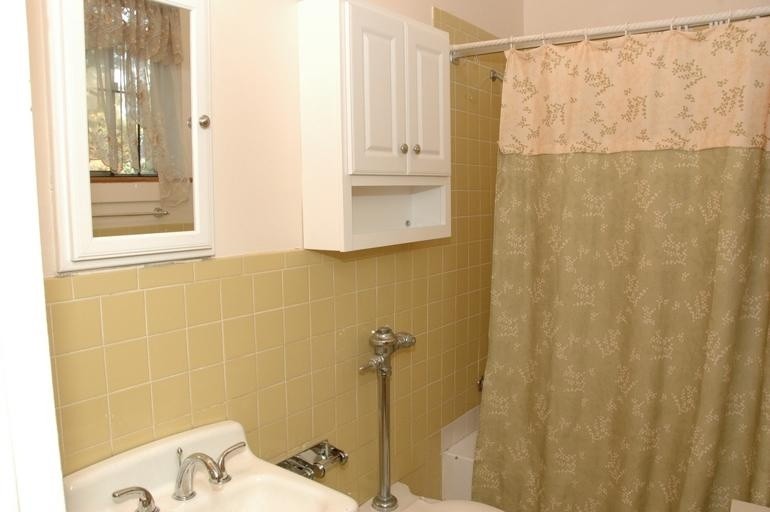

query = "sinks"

[{"left": 64, "top": 422, "right": 358, "bottom": 512}]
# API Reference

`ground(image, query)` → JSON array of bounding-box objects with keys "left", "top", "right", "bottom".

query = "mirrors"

[{"left": 77, "top": 0, "right": 200, "bottom": 243}]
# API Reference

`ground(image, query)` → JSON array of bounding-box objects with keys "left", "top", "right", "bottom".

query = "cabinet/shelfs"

[{"left": 297, "top": 0, "right": 453, "bottom": 255}]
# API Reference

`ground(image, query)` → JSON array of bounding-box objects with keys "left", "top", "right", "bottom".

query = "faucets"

[{"left": 174, "top": 450, "right": 224, "bottom": 499}]
